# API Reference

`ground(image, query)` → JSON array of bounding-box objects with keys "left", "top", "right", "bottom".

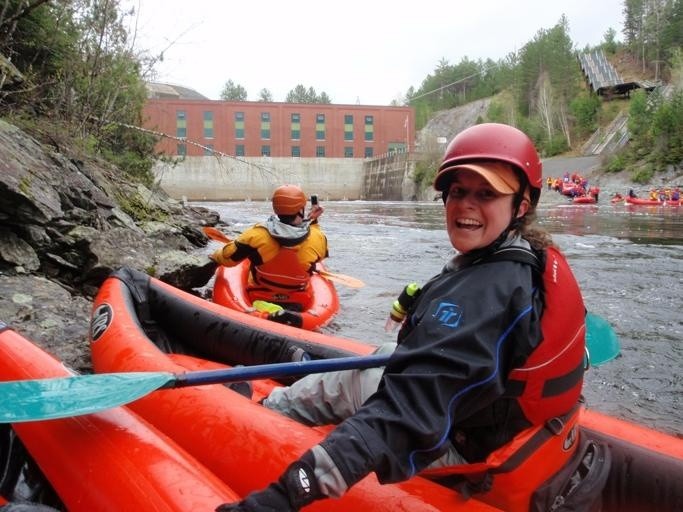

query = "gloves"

[{"left": 215, "top": 447, "right": 330, "bottom": 512}]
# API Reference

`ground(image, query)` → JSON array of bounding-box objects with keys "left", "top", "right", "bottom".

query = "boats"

[
  {"left": 612, "top": 196, "right": 682, "bottom": 206},
  {"left": 211, "top": 256, "right": 340, "bottom": 333},
  {"left": 89, "top": 256, "right": 683, "bottom": 512},
  {"left": 1, "top": 328, "right": 243, "bottom": 512},
  {"left": 561, "top": 181, "right": 596, "bottom": 203}
]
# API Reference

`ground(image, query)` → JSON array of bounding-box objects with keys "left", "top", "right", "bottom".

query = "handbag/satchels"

[{"left": 528, "top": 427, "right": 612, "bottom": 512}]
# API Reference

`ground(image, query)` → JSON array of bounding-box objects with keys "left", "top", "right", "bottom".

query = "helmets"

[
  {"left": 272, "top": 184, "right": 307, "bottom": 216},
  {"left": 433, "top": 122, "right": 542, "bottom": 203}
]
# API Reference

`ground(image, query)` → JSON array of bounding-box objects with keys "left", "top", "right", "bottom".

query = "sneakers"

[
  {"left": 225, "top": 364, "right": 253, "bottom": 400},
  {"left": 287, "top": 345, "right": 311, "bottom": 386}
]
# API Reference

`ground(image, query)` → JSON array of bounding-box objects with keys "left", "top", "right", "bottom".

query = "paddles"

[
  {"left": 202, "top": 224, "right": 364, "bottom": 291},
  {"left": 0, "top": 315, "right": 621, "bottom": 425}
]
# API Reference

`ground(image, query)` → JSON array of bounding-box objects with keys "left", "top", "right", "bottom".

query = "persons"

[
  {"left": 544, "top": 169, "right": 683, "bottom": 209},
  {"left": 209, "top": 122, "right": 586, "bottom": 509},
  {"left": 209, "top": 184, "right": 329, "bottom": 310}
]
